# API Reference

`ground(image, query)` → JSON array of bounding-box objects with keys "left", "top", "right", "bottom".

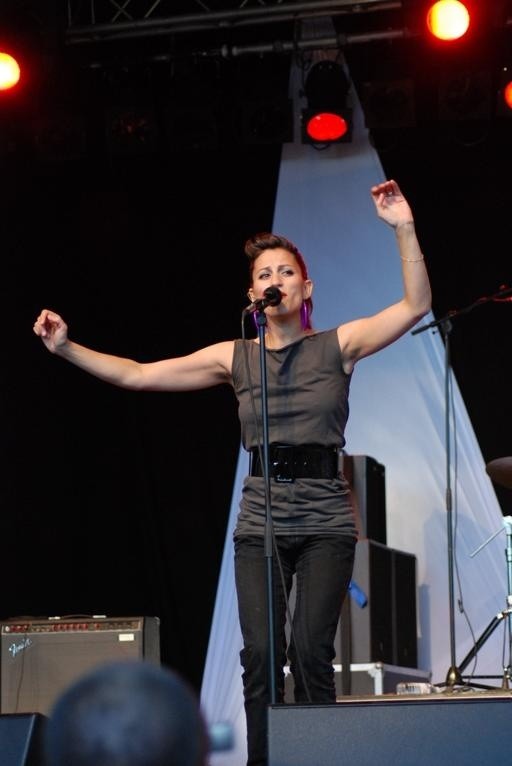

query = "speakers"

[
  {"left": 267, "top": 699, "right": 512, "bottom": 766},
  {"left": 284, "top": 455, "right": 418, "bottom": 669},
  {"left": 0, "top": 712, "right": 49, "bottom": 766},
  {"left": 0, "top": 616, "right": 161, "bottom": 716}
]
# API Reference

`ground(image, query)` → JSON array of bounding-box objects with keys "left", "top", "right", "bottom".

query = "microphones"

[{"left": 244, "top": 287, "right": 282, "bottom": 315}]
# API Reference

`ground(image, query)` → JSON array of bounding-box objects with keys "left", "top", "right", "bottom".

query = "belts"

[{"left": 246, "top": 443, "right": 349, "bottom": 483}]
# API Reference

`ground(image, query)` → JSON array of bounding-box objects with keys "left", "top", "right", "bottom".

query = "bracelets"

[{"left": 400, "top": 251, "right": 423, "bottom": 263}]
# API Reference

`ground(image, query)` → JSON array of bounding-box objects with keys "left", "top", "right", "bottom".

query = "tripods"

[
  {"left": 411, "top": 283, "right": 512, "bottom": 690},
  {"left": 458, "top": 514, "right": 512, "bottom": 690}
]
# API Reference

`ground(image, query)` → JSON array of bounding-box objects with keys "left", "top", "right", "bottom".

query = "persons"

[
  {"left": 33, "top": 178, "right": 433, "bottom": 765},
  {"left": 41, "top": 659, "right": 209, "bottom": 766}
]
